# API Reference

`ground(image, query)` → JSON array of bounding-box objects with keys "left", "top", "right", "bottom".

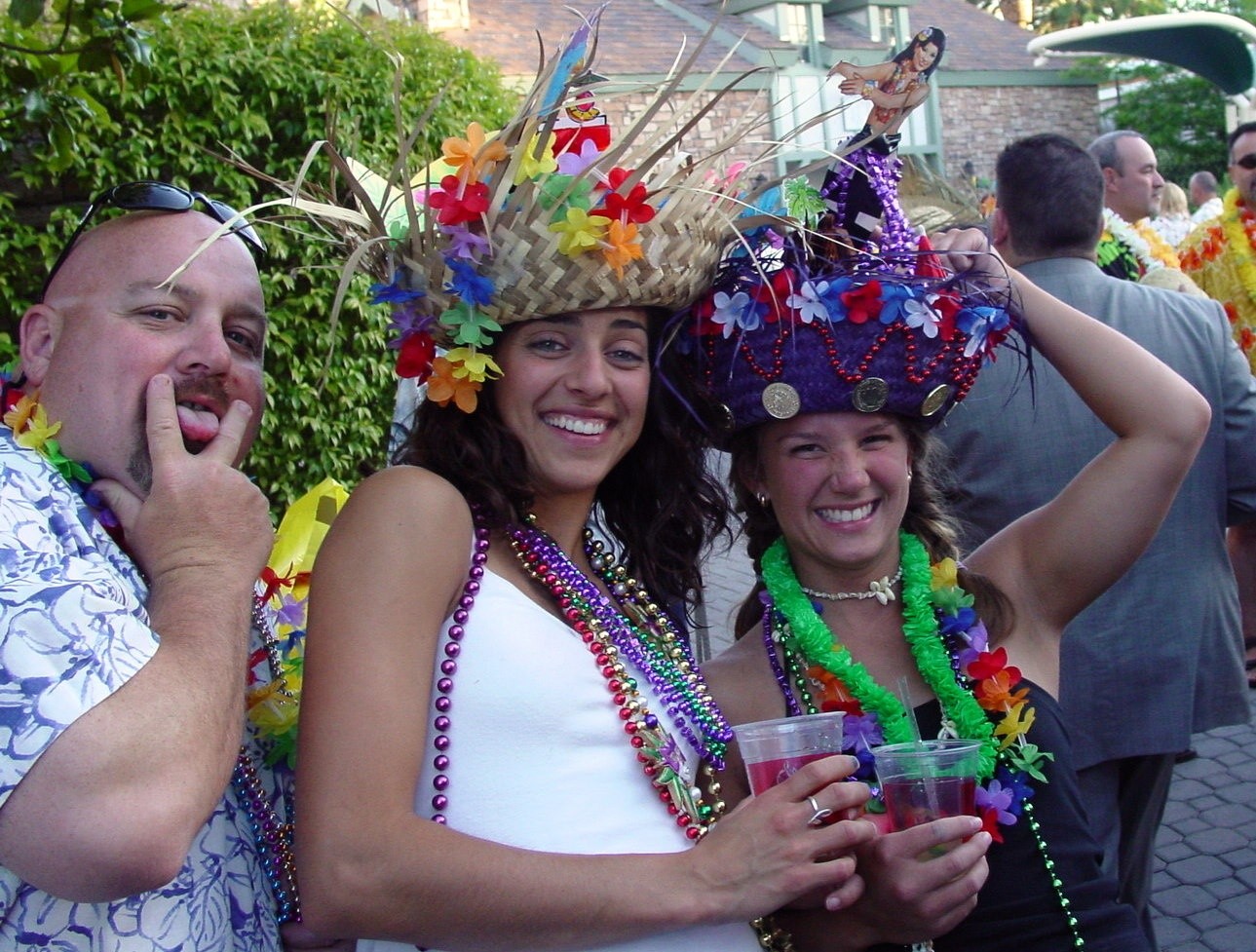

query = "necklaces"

[
  {"left": 1102, "top": 208, "right": 1182, "bottom": 273},
  {"left": 1236, "top": 196, "right": 1256, "bottom": 249},
  {"left": 876, "top": 58, "right": 927, "bottom": 123},
  {"left": 0, "top": 359, "right": 1083, "bottom": 952},
  {"left": 1223, "top": 184, "right": 1256, "bottom": 305}
]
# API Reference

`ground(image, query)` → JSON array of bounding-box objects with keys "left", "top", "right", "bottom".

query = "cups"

[
  {"left": 729, "top": 711, "right": 849, "bottom": 908},
  {"left": 870, "top": 738, "right": 981, "bottom": 880}
]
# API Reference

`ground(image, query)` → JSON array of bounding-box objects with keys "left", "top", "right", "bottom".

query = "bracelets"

[{"left": 863, "top": 80, "right": 875, "bottom": 100}]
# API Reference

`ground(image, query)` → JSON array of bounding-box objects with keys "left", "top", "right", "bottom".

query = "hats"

[
  {"left": 654, "top": 27, "right": 1049, "bottom": 451},
  {"left": 153, "top": 2, "right": 917, "bottom": 415}
]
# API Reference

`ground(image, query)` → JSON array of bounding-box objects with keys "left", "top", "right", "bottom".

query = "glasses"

[
  {"left": 1231, "top": 152, "right": 1256, "bottom": 171},
  {"left": 41, "top": 181, "right": 267, "bottom": 299}
]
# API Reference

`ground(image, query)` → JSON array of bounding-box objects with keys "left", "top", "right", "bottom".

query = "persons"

[
  {"left": 0, "top": 210, "right": 295, "bottom": 952},
  {"left": 917, "top": 119, "right": 1256, "bottom": 951},
  {"left": 808, "top": 26, "right": 946, "bottom": 276},
  {"left": 675, "top": 224, "right": 1211, "bottom": 952},
  {"left": 295, "top": 218, "right": 876, "bottom": 952}
]
